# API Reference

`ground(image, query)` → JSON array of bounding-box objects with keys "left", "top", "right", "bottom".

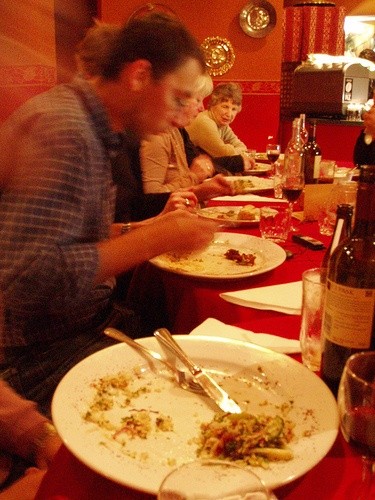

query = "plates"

[
  {"left": 246, "top": 151, "right": 280, "bottom": 160},
  {"left": 197, "top": 206, "right": 282, "bottom": 227},
  {"left": 137, "top": 232, "right": 286, "bottom": 280},
  {"left": 244, "top": 162, "right": 273, "bottom": 174},
  {"left": 239, "top": 0, "right": 276, "bottom": 38},
  {"left": 335, "top": 166, "right": 360, "bottom": 178},
  {"left": 203, "top": 176, "right": 275, "bottom": 194},
  {"left": 48, "top": 338, "right": 338, "bottom": 495}
]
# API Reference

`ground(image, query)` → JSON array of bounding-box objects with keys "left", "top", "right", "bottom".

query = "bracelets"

[{"left": 121, "top": 222, "right": 131, "bottom": 235}]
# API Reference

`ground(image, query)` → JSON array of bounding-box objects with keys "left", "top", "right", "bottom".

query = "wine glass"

[
  {"left": 282, "top": 175, "right": 304, "bottom": 230},
  {"left": 336, "top": 351, "right": 375, "bottom": 500},
  {"left": 266, "top": 143, "right": 281, "bottom": 167}
]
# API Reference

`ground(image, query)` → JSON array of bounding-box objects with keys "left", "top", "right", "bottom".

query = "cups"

[
  {"left": 156, "top": 461, "right": 278, "bottom": 500},
  {"left": 300, "top": 266, "right": 333, "bottom": 373},
  {"left": 301, "top": 181, "right": 360, "bottom": 235},
  {"left": 319, "top": 160, "right": 335, "bottom": 184}
]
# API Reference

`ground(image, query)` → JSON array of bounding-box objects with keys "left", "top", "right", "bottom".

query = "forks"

[{"left": 103, "top": 327, "right": 222, "bottom": 394}]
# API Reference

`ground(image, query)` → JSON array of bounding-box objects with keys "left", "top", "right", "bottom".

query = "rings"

[
  {"left": 185, "top": 199, "right": 190, "bottom": 206},
  {"left": 208, "top": 166, "right": 211, "bottom": 171}
]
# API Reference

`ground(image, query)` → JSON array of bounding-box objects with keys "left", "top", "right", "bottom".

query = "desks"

[{"left": 37, "top": 162, "right": 375, "bottom": 500}]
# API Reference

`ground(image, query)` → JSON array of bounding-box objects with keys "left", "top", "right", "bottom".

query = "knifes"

[{"left": 153, "top": 329, "right": 242, "bottom": 419}]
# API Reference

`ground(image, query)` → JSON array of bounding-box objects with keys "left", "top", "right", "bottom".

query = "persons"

[
  {"left": 362, "top": 104, "right": 375, "bottom": 141},
  {"left": 0, "top": 379, "right": 63, "bottom": 500},
  {"left": 0, "top": 13, "right": 257, "bottom": 421}
]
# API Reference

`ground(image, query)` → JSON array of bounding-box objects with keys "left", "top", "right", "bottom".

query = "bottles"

[
  {"left": 282, "top": 112, "right": 320, "bottom": 193},
  {"left": 322, "top": 159, "right": 375, "bottom": 389}
]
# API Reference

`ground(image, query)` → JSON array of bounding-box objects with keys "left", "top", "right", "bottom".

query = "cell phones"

[{"left": 293, "top": 234, "right": 324, "bottom": 249}]
